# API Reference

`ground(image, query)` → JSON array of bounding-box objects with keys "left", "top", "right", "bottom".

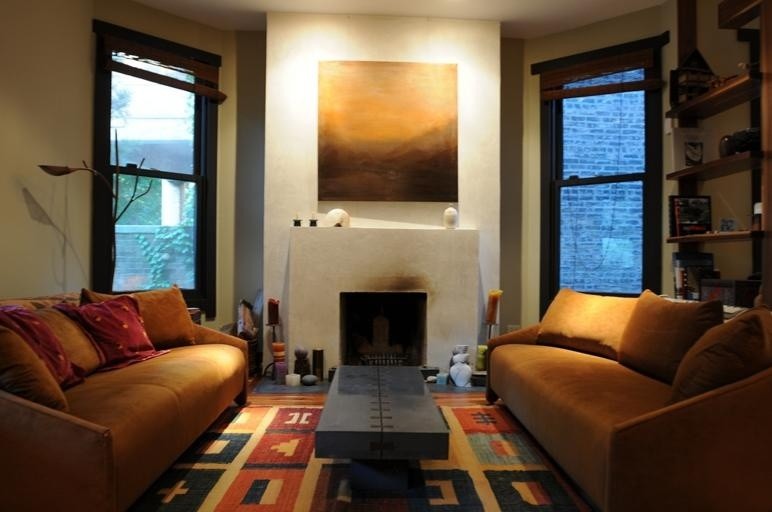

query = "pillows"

[
  {"left": 0, "top": 284, "right": 195, "bottom": 413},
  {"left": 536, "top": 288, "right": 772, "bottom": 406}
]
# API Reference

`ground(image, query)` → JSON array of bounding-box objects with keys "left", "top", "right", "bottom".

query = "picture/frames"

[
  {"left": 669, "top": 195, "right": 712, "bottom": 237},
  {"left": 683, "top": 140, "right": 706, "bottom": 168}
]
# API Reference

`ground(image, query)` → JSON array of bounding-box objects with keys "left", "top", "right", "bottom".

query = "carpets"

[{"left": 125, "top": 391, "right": 597, "bottom": 512}]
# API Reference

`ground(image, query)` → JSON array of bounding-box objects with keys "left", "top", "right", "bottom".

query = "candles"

[
  {"left": 486, "top": 288, "right": 503, "bottom": 322},
  {"left": 268, "top": 297, "right": 279, "bottom": 325}
]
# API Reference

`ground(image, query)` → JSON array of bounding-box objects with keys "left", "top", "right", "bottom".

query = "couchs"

[
  {"left": 0, "top": 288, "right": 249, "bottom": 511},
  {"left": 486, "top": 288, "right": 772, "bottom": 511}
]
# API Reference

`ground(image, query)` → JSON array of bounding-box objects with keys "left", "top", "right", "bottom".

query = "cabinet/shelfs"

[{"left": 666, "top": 1, "right": 772, "bottom": 306}]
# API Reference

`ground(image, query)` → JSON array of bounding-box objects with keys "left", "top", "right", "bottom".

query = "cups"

[{"left": 275, "top": 362, "right": 289, "bottom": 384}]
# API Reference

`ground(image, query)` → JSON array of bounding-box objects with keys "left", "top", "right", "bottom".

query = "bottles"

[
  {"left": 443, "top": 204, "right": 458, "bottom": 229},
  {"left": 313, "top": 349, "right": 324, "bottom": 383}
]
